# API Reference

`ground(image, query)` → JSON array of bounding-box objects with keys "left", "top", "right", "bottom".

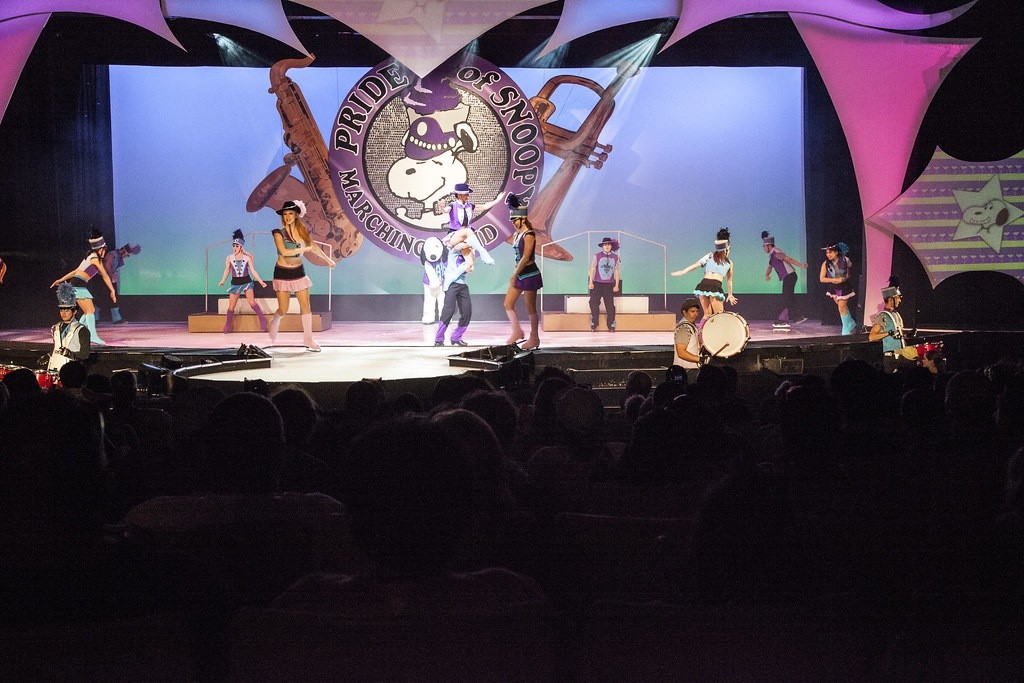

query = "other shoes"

[
  {"left": 434, "top": 340, "right": 467, "bottom": 347},
  {"left": 772, "top": 318, "right": 808, "bottom": 327},
  {"left": 591, "top": 319, "right": 615, "bottom": 332}
]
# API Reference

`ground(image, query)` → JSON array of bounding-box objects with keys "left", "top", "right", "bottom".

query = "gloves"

[
  {"left": 700, "top": 356, "right": 712, "bottom": 364},
  {"left": 36, "top": 346, "right": 71, "bottom": 366},
  {"left": 889, "top": 328, "right": 917, "bottom": 340}
]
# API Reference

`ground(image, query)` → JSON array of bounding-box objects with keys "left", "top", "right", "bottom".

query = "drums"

[
  {"left": 0, "top": 364, "right": 33, "bottom": 382},
  {"left": 700, "top": 311, "right": 751, "bottom": 359},
  {"left": 915, "top": 341, "right": 943, "bottom": 357},
  {"left": 34, "top": 370, "right": 63, "bottom": 390}
]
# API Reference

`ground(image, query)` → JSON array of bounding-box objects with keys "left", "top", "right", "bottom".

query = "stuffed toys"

[{"left": 420, "top": 236, "right": 450, "bottom": 324}]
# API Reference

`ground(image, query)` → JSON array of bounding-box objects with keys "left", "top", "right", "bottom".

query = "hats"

[
  {"left": 232, "top": 228, "right": 245, "bottom": 246},
  {"left": 57, "top": 283, "right": 77, "bottom": 308},
  {"left": 821, "top": 240, "right": 839, "bottom": 250},
  {"left": 762, "top": 231, "right": 774, "bottom": 244},
  {"left": 714, "top": 229, "right": 730, "bottom": 253},
  {"left": 88, "top": 224, "right": 107, "bottom": 250},
  {"left": 454, "top": 184, "right": 473, "bottom": 194},
  {"left": 276, "top": 201, "right": 301, "bottom": 214},
  {"left": 598, "top": 238, "right": 614, "bottom": 247},
  {"left": 681, "top": 297, "right": 700, "bottom": 314},
  {"left": 122, "top": 243, "right": 142, "bottom": 255},
  {"left": 880, "top": 275, "right": 901, "bottom": 300},
  {"left": 506, "top": 193, "right": 528, "bottom": 221}
]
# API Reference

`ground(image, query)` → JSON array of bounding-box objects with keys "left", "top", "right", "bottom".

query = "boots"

[
  {"left": 840, "top": 308, "right": 856, "bottom": 336},
  {"left": 223, "top": 308, "right": 234, "bottom": 334},
  {"left": 79, "top": 313, "right": 106, "bottom": 346},
  {"left": 269, "top": 311, "right": 286, "bottom": 343},
  {"left": 697, "top": 318, "right": 707, "bottom": 344},
  {"left": 95, "top": 306, "right": 128, "bottom": 327},
  {"left": 506, "top": 310, "right": 540, "bottom": 351},
  {"left": 252, "top": 303, "right": 267, "bottom": 331},
  {"left": 467, "top": 234, "right": 495, "bottom": 264},
  {"left": 302, "top": 312, "right": 321, "bottom": 352}
]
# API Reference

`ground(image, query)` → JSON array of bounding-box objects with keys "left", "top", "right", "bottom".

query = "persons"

[
  {"left": 35, "top": 281, "right": 91, "bottom": 385},
  {"left": 50, "top": 235, "right": 117, "bottom": 346},
  {"left": 92, "top": 242, "right": 142, "bottom": 326},
  {"left": 760, "top": 231, "right": 809, "bottom": 328},
  {"left": 503, "top": 193, "right": 543, "bottom": 350},
  {"left": 589, "top": 237, "right": 620, "bottom": 332},
  {"left": 669, "top": 226, "right": 738, "bottom": 344},
  {"left": 0, "top": 360, "right": 1024, "bottom": 604},
  {"left": 267, "top": 199, "right": 336, "bottom": 352},
  {"left": 819, "top": 241, "right": 857, "bottom": 336},
  {"left": 435, "top": 183, "right": 507, "bottom": 347},
  {"left": 672, "top": 298, "right": 715, "bottom": 384},
  {"left": 217, "top": 228, "right": 269, "bottom": 334},
  {"left": 922, "top": 349, "right": 946, "bottom": 374},
  {"left": 868, "top": 286, "right": 917, "bottom": 373}
]
